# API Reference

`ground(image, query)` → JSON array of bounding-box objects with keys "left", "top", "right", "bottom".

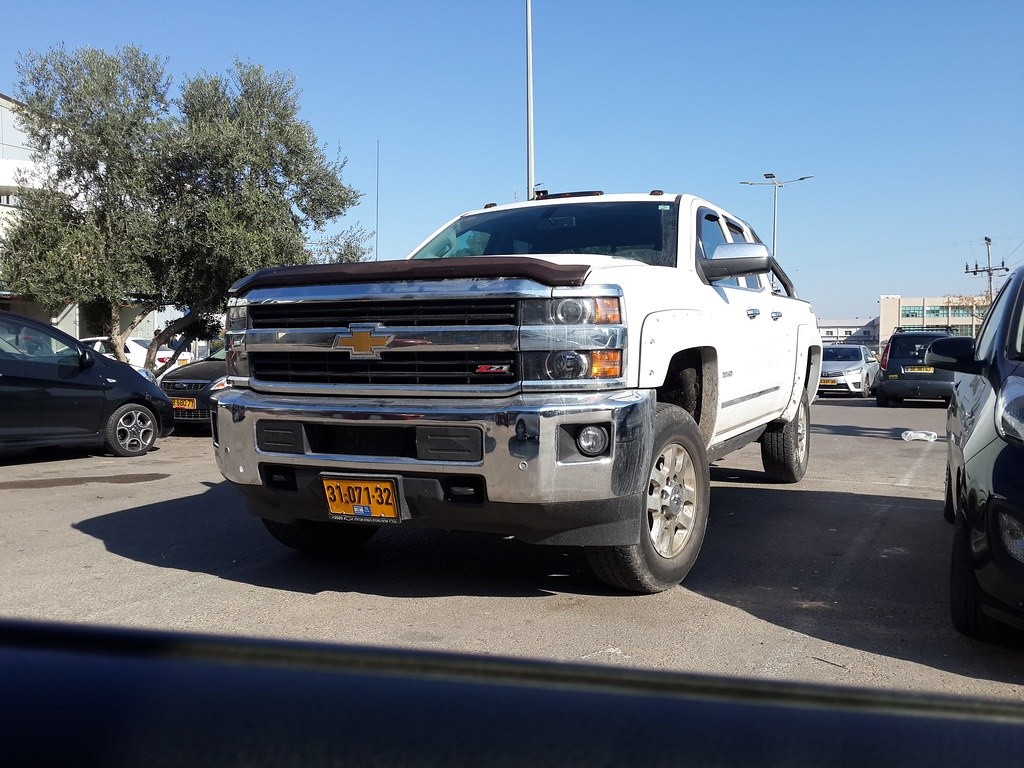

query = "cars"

[
  {"left": 817, "top": 345, "right": 880, "bottom": 398},
  {"left": 0, "top": 310, "right": 176, "bottom": 457},
  {"left": 159, "top": 344, "right": 232, "bottom": 424},
  {"left": 1, "top": 332, "right": 56, "bottom": 357},
  {"left": 923, "top": 264, "right": 1024, "bottom": 642},
  {"left": 53, "top": 336, "right": 195, "bottom": 385}
]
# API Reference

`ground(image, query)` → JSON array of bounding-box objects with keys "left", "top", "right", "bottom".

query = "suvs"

[
  {"left": 209, "top": 190, "right": 826, "bottom": 594},
  {"left": 877, "top": 326, "right": 955, "bottom": 410}
]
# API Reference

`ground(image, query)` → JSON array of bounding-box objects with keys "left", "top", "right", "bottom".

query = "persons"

[{"left": 153, "top": 329, "right": 191, "bottom": 352}]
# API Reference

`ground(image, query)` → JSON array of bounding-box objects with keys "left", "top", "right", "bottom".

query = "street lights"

[{"left": 740, "top": 172, "right": 815, "bottom": 288}]
